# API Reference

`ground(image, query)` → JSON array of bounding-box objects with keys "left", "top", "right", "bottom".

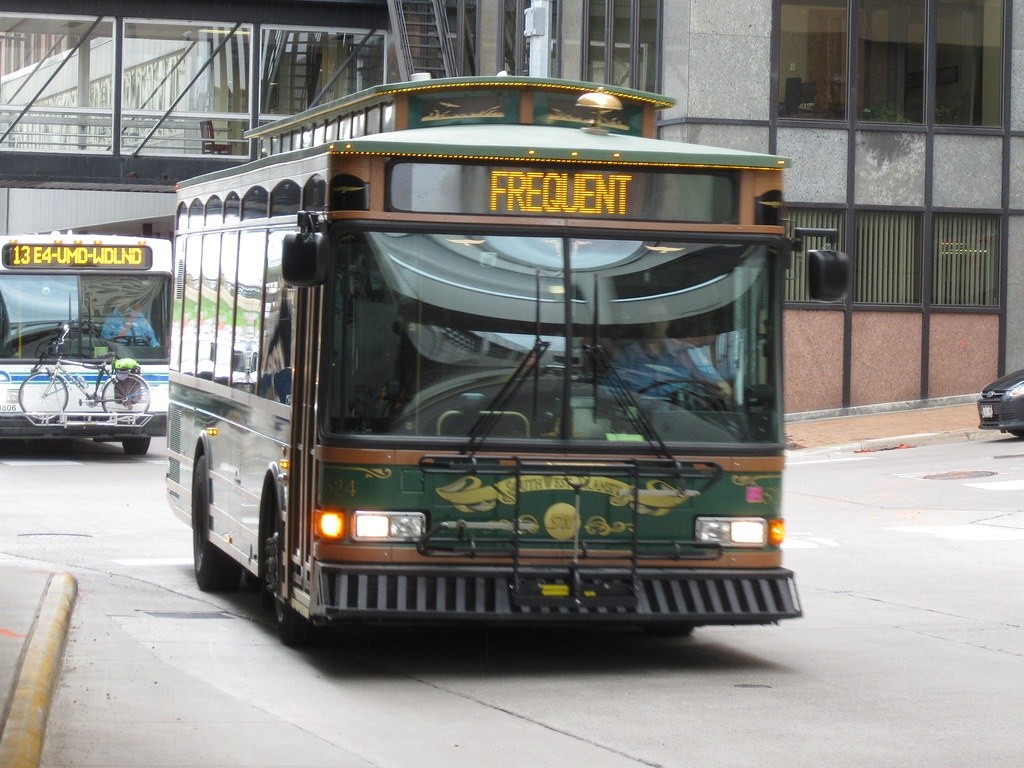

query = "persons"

[
  {"left": 605, "top": 297, "right": 733, "bottom": 410},
  {"left": 97, "top": 294, "right": 160, "bottom": 347}
]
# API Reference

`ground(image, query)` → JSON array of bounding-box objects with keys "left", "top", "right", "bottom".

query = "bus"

[
  {"left": 0, "top": 230, "right": 174, "bottom": 456},
  {"left": 163, "top": 71, "right": 849, "bottom": 650}
]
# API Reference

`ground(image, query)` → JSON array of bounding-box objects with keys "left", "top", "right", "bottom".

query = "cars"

[{"left": 978, "top": 368, "right": 1024, "bottom": 439}]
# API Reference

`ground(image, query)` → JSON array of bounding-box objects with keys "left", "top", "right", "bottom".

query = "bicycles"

[{"left": 17, "top": 336, "right": 152, "bottom": 423}]
